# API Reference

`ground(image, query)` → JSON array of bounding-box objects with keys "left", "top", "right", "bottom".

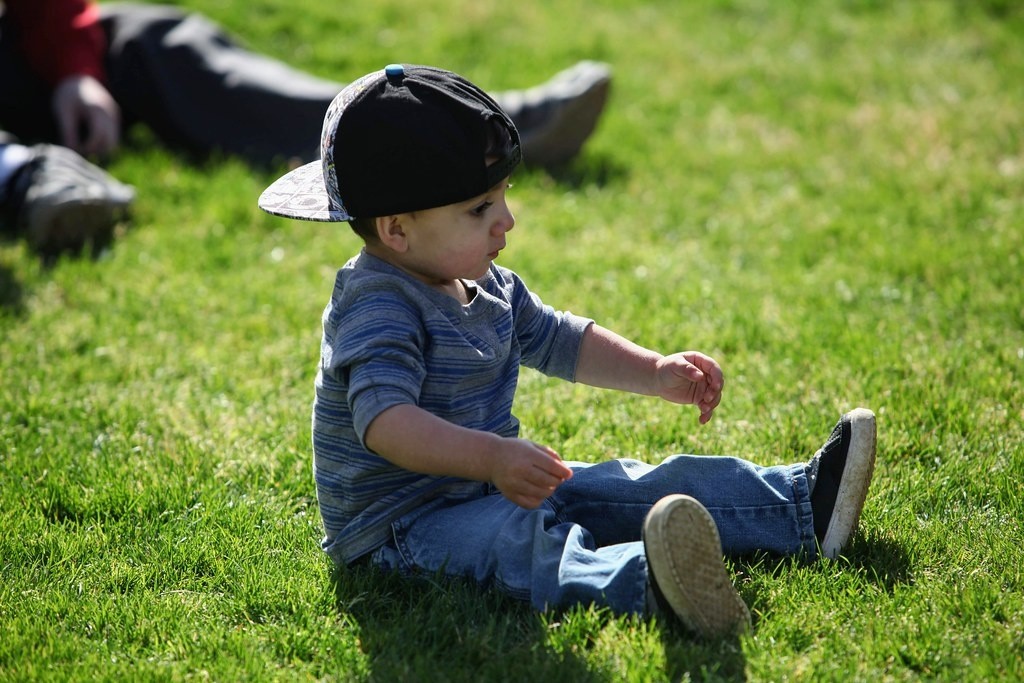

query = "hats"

[{"left": 259, "top": 62, "right": 523, "bottom": 221}]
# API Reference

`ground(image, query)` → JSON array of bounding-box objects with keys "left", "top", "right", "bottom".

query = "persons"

[
  {"left": 258, "top": 64, "right": 878, "bottom": 639},
  {"left": 0, "top": 0, "right": 614, "bottom": 265}
]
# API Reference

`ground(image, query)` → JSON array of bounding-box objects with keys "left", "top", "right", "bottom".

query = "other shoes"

[
  {"left": 485, "top": 60, "right": 611, "bottom": 172},
  {"left": 26, "top": 143, "right": 141, "bottom": 256}
]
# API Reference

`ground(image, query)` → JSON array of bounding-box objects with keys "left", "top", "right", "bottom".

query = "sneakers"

[
  {"left": 642, "top": 496, "right": 756, "bottom": 640},
  {"left": 807, "top": 406, "right": 877, "bottom": 561}
]
